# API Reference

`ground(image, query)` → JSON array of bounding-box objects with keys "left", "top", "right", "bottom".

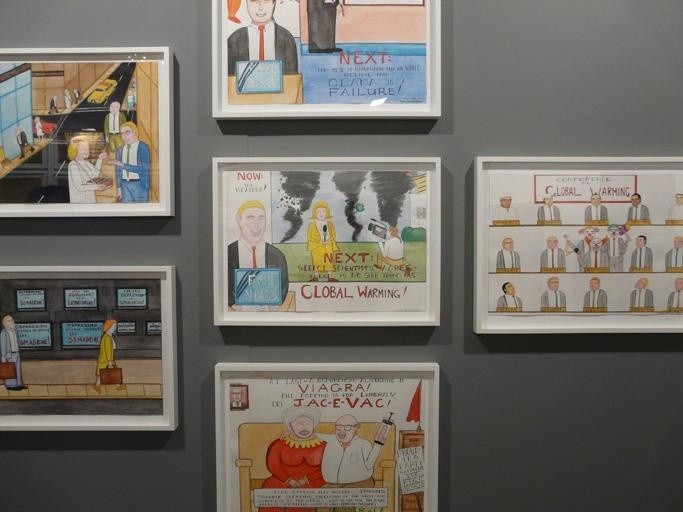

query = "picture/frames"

[
  {"left": 212, "top": 157, "right": 442, "bottom": 326},
  {"left": 0, "top": 47, "right": 175, "bottom": 217},
  {"left": 214, "top": 363, "right": 439, "bottom": 512},
  {"left": 212, "top": 0, "right": 442, "bottom": 120},
  {"left": 473, "top": 156, "right": 682, "bottom": 334},
  {"left": 0, "top": 265, "right": 179, "bottom": 432}
]
